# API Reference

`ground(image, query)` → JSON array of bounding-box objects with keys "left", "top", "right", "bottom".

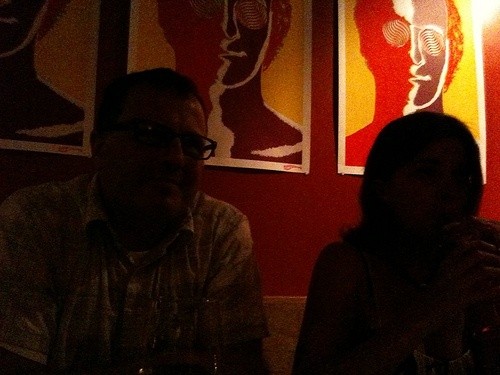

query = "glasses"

[{"left": 106, "top": 118, "right": 217, "bottom": 160}]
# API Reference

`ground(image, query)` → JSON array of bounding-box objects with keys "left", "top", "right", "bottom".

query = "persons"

[
  {"left": 290, "top": 112, "right": 500, "bottom": 375},
  {"left": 0, "top": 68, "right": 271, "bottom": 375}
]
